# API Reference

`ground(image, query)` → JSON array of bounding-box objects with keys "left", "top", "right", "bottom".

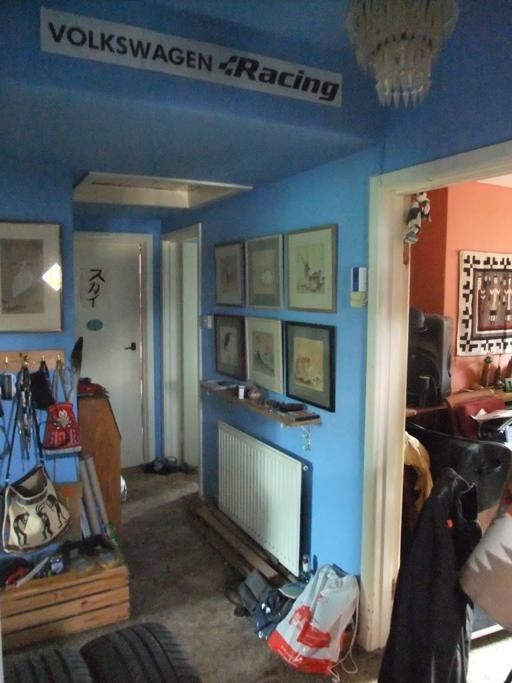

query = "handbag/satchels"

[
  {"left": 268, "top": 563, "right": 358, "bottom": 674},
  {"left": 44, "top": 401, "right": 80, "bottom": 451},
  {"left": 29, "top": 371, "right": 55, "bottom": 412},
  {"left": 0, "top": 464, "right": 74, "bottom": 555}
]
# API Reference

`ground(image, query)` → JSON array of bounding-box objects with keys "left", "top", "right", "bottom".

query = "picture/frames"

[
  {"left": 0, "top": 219, "right": 65, "bottom": 333},
  {"left": 210, "top": 222, "right": 340, "bottom": 414},
  {"left": 457, "top": 248, "right": 511, "bottom": 358}
]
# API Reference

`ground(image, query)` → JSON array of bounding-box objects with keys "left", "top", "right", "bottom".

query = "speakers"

[{"left": 408, "top": 308, "right": 452, "bottom": 401}]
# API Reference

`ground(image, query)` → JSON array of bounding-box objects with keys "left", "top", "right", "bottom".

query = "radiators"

[{"left": 215, "top": 416, "right": 311, "bottom": 579}]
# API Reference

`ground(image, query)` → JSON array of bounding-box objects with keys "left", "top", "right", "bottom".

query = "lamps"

[{"left": 342, "top": 0, "right": 461, "bottom": 110}]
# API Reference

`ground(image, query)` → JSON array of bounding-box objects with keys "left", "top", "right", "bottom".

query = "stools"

[{"left": 2, "top": 554, "right": 132, "bottom": 657}]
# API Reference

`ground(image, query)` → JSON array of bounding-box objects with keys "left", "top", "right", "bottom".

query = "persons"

[{"left": 403, "top": 192, "right": 429, "bottom": 250}]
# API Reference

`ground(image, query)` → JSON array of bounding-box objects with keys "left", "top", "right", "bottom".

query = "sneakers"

[{"left": 70, "top": 536, "right": 122, "bottom": 573}]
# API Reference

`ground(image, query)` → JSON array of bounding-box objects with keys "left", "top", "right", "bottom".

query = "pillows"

[{"left": 52, "top": 480, "right": 84, "bottom": 544}]
